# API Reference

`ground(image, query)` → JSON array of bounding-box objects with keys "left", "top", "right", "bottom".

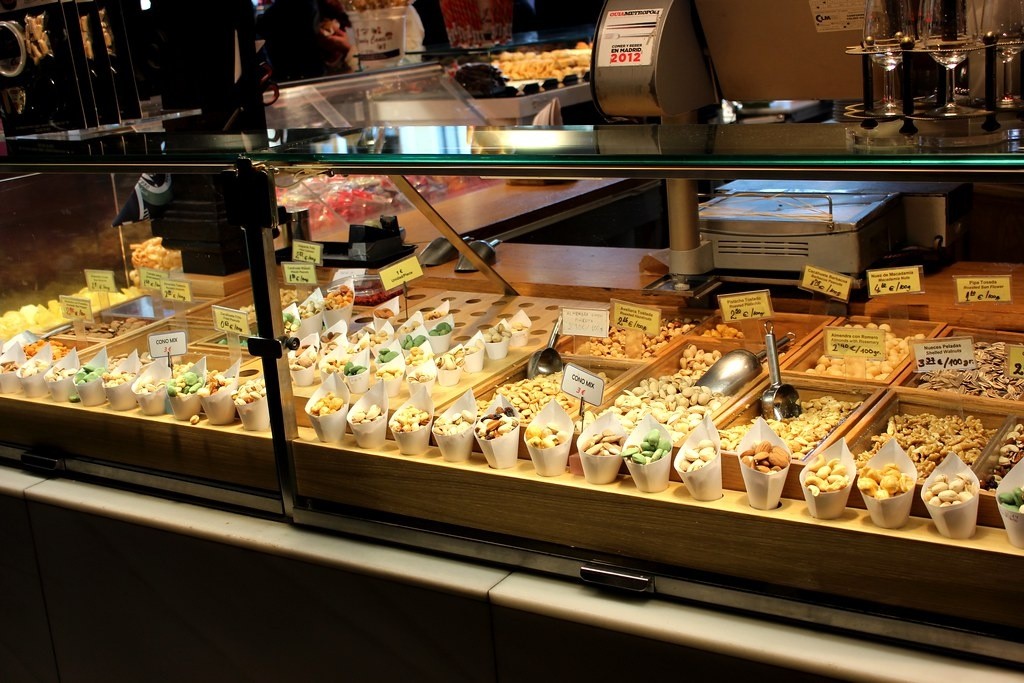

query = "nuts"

[{"left": 0, "top": 285, "right": 1024, "bottom": 513}]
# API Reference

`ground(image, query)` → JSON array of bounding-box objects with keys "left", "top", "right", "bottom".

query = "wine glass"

[
  {"left": 861, "top": 0, "right": 915, "bottom": 116},
  {"left": 916, "top": 0, "right": 978, "bottom": 117},
  {"left": 980, "top": 0, "right": 1024, "bottom": 109}
]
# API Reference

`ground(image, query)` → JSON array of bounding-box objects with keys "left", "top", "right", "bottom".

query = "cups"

[{"left": 345, "top": 6, "right": 408, "bottom": 64}]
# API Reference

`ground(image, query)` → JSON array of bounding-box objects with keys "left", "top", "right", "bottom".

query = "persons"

[{"left": 319, "top": 0, "right": 424, "bottom": 73}]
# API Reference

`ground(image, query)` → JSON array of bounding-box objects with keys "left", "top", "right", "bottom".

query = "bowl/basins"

[{"left": 532, "top": 97, "right": 563, "bottom": 126}]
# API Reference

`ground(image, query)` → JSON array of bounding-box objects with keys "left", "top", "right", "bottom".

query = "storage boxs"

[{"left": 0, "top": 279, "right": 1024, "bottom": 531}]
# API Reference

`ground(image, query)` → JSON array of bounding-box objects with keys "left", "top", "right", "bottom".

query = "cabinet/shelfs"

[
  {"left": 132, "top": 60, "right": 507, "bottom": 133},
  {"left": 0, "top": 156, "right": 1024, "bottom": 683}
]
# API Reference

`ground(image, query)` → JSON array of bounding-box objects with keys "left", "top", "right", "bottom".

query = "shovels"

[
  {"left": 694, "top": 332, "right": 796, "bottom": 398},
  {"left": 453, "top": 239, "right": 500, "bottom": 272},
  {"left": 760, "top": 320, "right": 802, "bottom": 421},
  {"left": 417, "top": 236, "right": 471, "bottom": 266},
  {"left": 527, "top": 315, "right": 563, "bottom": 381}
]
